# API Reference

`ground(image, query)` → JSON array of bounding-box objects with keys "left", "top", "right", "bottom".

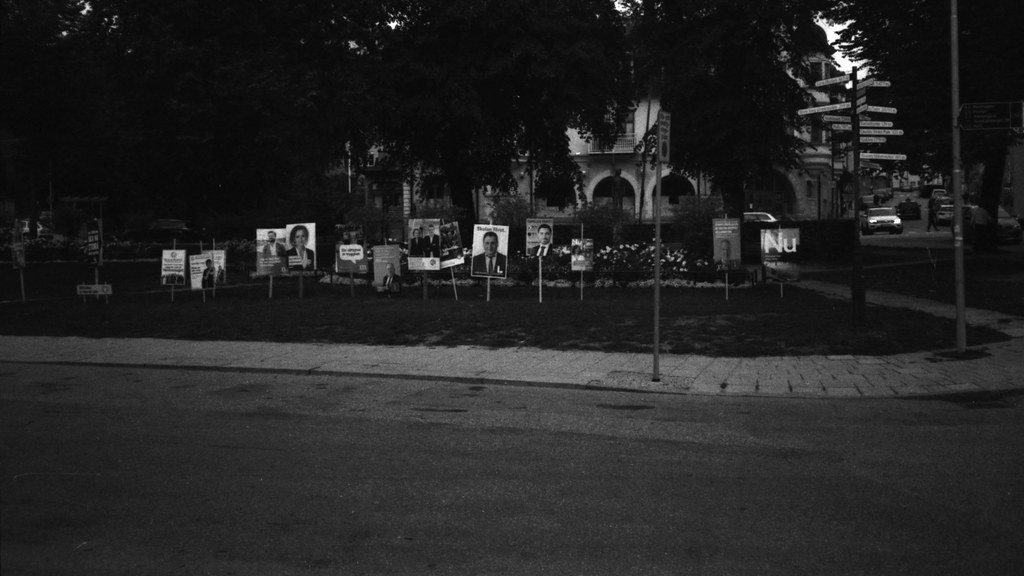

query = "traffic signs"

[{"left": 798, "top": 74, "right": 907, "bottom": 160}]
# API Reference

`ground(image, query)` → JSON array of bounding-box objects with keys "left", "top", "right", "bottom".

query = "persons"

[
  {"left": 716, "top": 240, "right": 740, "bottom": 269},
  {"left": 383, "top": 224, "right": 586, "bottom": 292},
  {"left": 873, "top": 190, "right": 994, "bottom": 252},
  {"left": 201, "top": 225, "right": 314, "bottom": 289},
  {"left": 612, "top": 168, "right": 624, "bottom": 209}
]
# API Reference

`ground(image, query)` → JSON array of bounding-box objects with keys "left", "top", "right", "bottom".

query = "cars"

[
  {"left": 931, "top": 188, "right": 970, "bottom": 226},
  {"left": 861, "top": 207, "right": 903, "bottom": 235},
  {"left": 861, "top": 187, "right": 893, "bottom": 207},
  {"left": 894, "top": 199, "right": 921, "bottom": 220},
  {"left": 951, "top": 205, "right": 1022, "bottom": 243}
]
